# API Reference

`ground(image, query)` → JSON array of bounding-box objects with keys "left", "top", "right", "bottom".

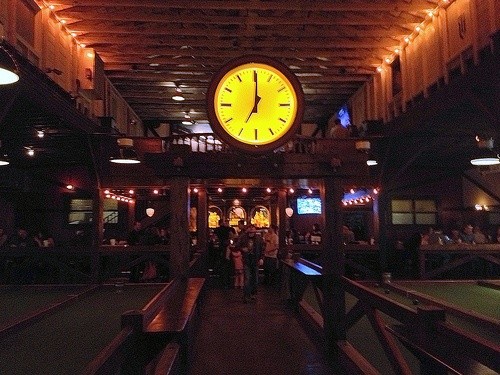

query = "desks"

[
  {"left": 0, "top": 282, "right": 167, "bottom": 375},
  {"left": 353, "top": 277, "right": 500, "bottom": 348}
]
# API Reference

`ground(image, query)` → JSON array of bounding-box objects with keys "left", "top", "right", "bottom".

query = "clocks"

[{"left": 204, "top": 53, "right": 306, "bottom": 158}]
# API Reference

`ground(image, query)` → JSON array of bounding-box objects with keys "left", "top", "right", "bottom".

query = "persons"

[
  {"left": 420, "top": 224, "right": 500, "bottom": 246},
  {"left": 214, "top": 218, "right": 279, "bottom": 303},
  {"left": 0, "top": 226, "right": 56, "bottom": 248},
  {"left": 330, "top": 119, "right": 348, "bottom": 138},
  {"left": 304, "top": 224, "right": 321, "bottom": 245},
  {"left": 126, "top": 221, "right": 171, "bottom": 279}
]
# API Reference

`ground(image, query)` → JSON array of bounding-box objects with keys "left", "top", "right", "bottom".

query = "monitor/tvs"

[
  {"left": 296, "top": 197, "right": 322, "bottom": 215},
  {"left": 337, "top": 105, "right": 352, "bottom": 128}
]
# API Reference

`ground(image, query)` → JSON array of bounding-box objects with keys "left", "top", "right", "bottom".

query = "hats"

[{"left": 245, "top": 227, "right": 258, "bottom": 233}]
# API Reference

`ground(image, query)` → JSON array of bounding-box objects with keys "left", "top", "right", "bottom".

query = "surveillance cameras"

[{"left": 53, "top": 68, "right": 63, "bottom": 76}]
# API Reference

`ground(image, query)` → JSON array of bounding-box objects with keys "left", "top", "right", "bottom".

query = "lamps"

[
  {"left": 104, "top": 190, "right": 134, "bottom": 204},
  {"left": 342, "top": 194, "right": 372, "bottom": 206},
  {"left": 45, "top": 68, "right": 64, "bottom": 76},
  {"left": 285, "top": 202, "right": 294, "bottom": 218},
  {"left": 47, "top": 4, "right": 93, "bottom": 58},
  {"left": 470, "top": 130, "right": 500, "bottom": 166},
  {"left": 376, "top": 0, "right": 450, "bottom": 73},
  {"left": 0, "top": 44, "right": 20, "bottom": 86},
  {"left": 146, "top": 200, "right": 155, "bottom": 218}
]
